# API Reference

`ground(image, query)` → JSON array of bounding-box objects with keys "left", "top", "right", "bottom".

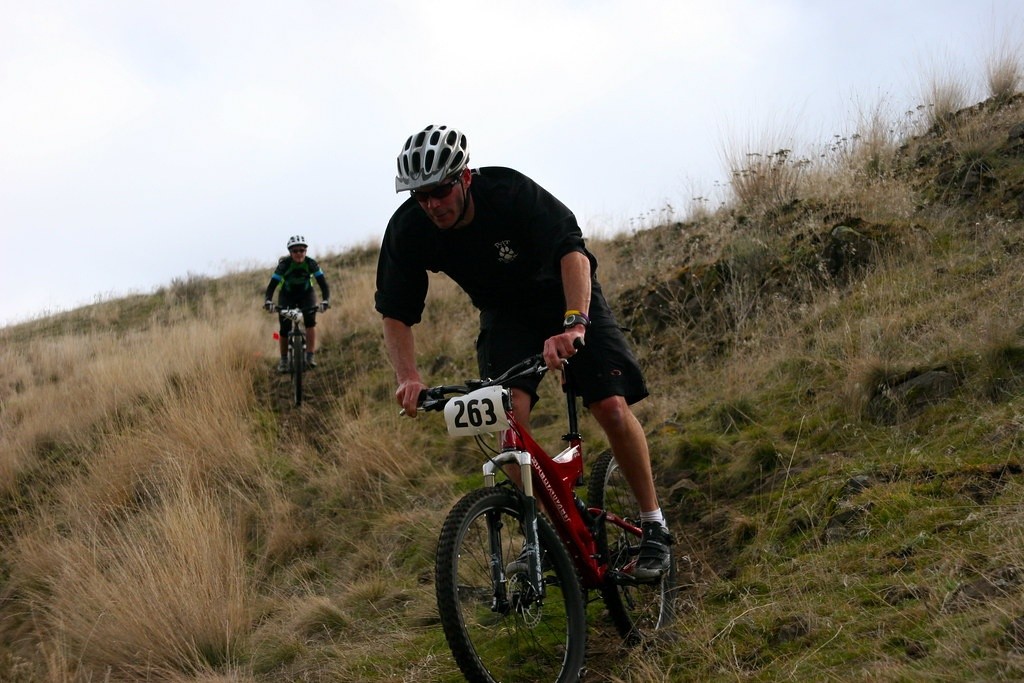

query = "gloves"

[
  {"left": 264, "top": 300, "right": 277, "bottom": 314},
  {"left": 318, "top": 300, "right": 329, "bottom": 314}
]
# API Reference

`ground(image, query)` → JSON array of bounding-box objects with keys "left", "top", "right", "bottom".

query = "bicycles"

[
  {"left": 262, "top": 302, "right": 332, "bottom": 408},
  {"left": 397, "top": 335, "right": 679, "bottom": 683}
]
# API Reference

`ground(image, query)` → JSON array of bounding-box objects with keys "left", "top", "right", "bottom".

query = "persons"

[
  {"left": 375, "top": 125, "right": 671, "bottom": 580},
  {"left": 265, "top": 236, "right": 330, "bottom": 372}
]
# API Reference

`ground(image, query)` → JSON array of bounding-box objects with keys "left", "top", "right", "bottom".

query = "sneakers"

[
  {"left": 305, "top": 352, "right": 317, "bottom": 367},
  {"left": 634, "top": 517, "right": 671, "bottom": 579},
  {"left": 506, "top": 533, "right": 543, "bottom": 578},
  {"left": 278, "top": 356, "right": 288, "bottom": 372}
]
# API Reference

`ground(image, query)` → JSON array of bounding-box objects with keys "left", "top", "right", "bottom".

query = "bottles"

[{"left": 572, "top": 491, "right": 594, "bottom": 527}]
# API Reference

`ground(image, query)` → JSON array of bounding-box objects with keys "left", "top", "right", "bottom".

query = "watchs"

[{"left": 564, "top": 315, "right": 587, "bottom": 327}]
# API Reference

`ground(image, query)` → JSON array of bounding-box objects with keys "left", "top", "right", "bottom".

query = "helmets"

[
  {"left": 394, "top": 124, "right": 470, "bottom": 193},
  {"left": 287, "top": 235, "right": 308, "bottom": 249}
]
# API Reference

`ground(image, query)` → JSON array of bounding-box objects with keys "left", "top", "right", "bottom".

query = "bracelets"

[{"left": 565, "top": 310, "right": 589, "bottom": 321}]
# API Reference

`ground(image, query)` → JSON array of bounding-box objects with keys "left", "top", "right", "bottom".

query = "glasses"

[
  {"left": 290, "top": 248, "right": 305, "bottom": 254},
  {"left": 409, "top": 164, "right": 466, "bottom": 202}
]
